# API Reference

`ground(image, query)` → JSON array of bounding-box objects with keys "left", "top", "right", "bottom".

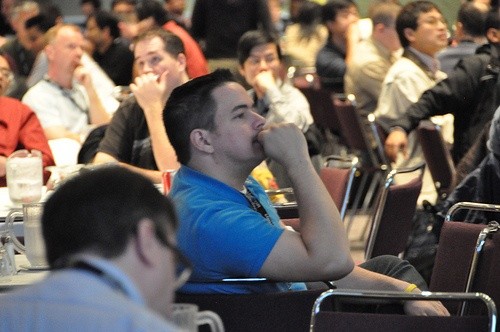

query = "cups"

[{"left": 170, "top": 302, "right": 225, "bottom": 332}]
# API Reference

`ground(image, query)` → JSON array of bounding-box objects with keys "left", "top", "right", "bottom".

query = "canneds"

[{"left": 161, "top": 169, "right": 178, "bottom": 195}]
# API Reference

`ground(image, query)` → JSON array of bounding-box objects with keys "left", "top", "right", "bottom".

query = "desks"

[
  {"left": 0, "top": 253, "right": 51, "bottom": 288},
  {"left": 0, "top": 180, "right": 168, "bottom": 235}
]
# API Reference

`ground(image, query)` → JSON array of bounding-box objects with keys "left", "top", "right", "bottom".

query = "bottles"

[{"left": 0, "top": 235, "right": 17, "bottom": 282}]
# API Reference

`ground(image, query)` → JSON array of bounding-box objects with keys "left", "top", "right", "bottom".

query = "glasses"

[{"left": 152, "top": 224, "right": 193, "bottom": 288}]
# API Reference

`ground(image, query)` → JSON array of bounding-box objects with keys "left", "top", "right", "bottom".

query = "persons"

[
  {"left": 0, "top": 165, "right": 181, "bottom": 332},
  {"left": 166, "top": 67, "right": 450, "bottom": 317},
  {"left": 0, "top": 0, "right": 499, "bottom": 189}
]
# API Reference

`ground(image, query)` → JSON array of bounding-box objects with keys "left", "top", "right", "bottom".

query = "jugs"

[
  {"left": 5, "top": 202, "right": 50, "bottom": 266},
  {"left": 6, "top": 148, "right": 43, "bottom": 204}
]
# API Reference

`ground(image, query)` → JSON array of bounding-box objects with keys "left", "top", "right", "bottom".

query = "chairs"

[{"left": 176, "top": 72, "right": 500, "bottom": 332}]
